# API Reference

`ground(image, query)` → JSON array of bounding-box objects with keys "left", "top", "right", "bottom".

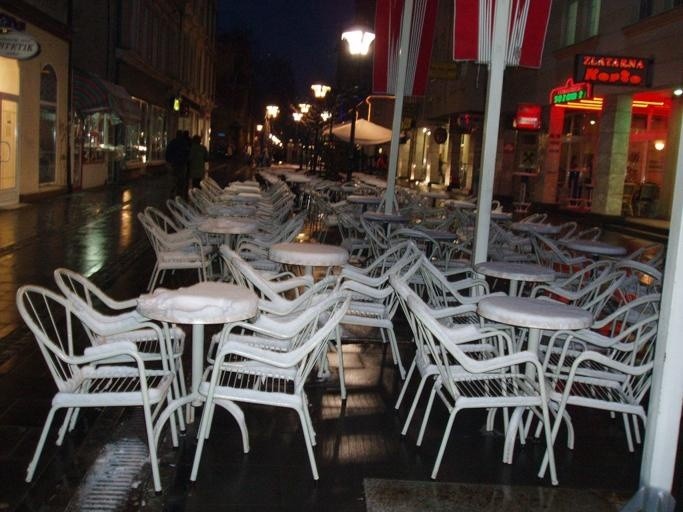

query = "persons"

[{"left": 166, "top": 130, "right": 208, "bottom": 201}]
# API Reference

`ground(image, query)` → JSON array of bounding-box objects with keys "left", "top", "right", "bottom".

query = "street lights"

[
  {"left": 260, "top": 79, "right": 333, "bottom": 176},
  {"left": 337, "top": 18, "right": 379, "bottom": 185}
]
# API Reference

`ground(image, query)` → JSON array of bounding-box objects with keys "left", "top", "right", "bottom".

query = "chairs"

[
  {"left": 523, "top": 313, "right": 661, "bottom": 479},
  {"left": 54, "top": 268, "right": 188, "bottom": 435},
  {"left": 190, "top": 291, "right": 351, "bottom": 481},
  {"left": 387, "top": 274, "right": 509, "bottom": 436},
  {"left": 539, "top": 293, "right": 661, "bottom": 420},
  {"left": 206, "top": 275, "right": 342, "bottom": 436},
  {"left": 135, "top": 167, "right": 667, "bottom": 327},
  {"left": 336, "top": 251, "right": 424, "bottom": 380},
  {"left": 15, "top": 285, "right": 178, "bottom": 492},
  {"left": 407, "top": 297, "right": 557, "bottom": 486}
]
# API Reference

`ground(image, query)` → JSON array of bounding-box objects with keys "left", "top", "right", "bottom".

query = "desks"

[
  {"left": 476, "top": 296, "right": 594, "bottom": 465},
  {"left": 138, "top": 279, "right": 259, "bottom": 463}
]
